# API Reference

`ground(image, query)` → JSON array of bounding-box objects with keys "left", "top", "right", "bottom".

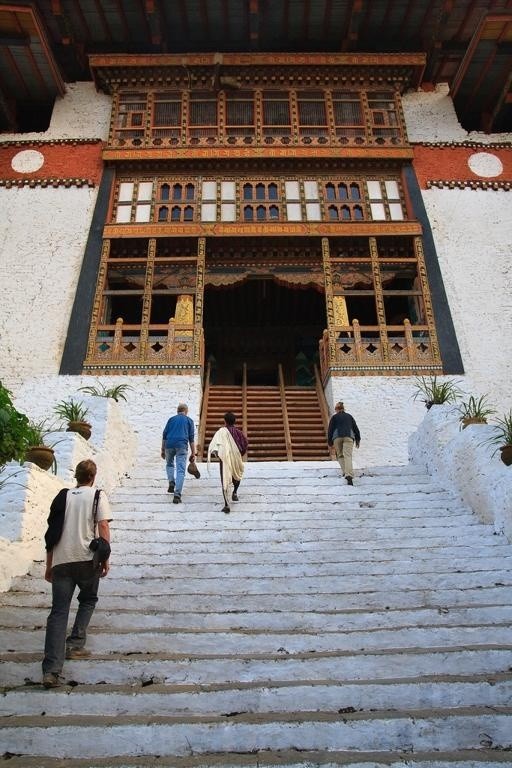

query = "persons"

[
  {"left": 328, "top": 402, "right": 361, "bottom": 486},
  {"left": 42, "top": 459, "right": 114, "bottom": 685},
  {"left": 161, "top": 403, "right": 195, "bottom": 504},
  {"left": 213, "top": 413, "right": 248, "bottom": 514}
]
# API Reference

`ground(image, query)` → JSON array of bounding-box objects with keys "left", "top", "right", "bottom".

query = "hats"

[
  {"left": 92, "top": 537, "right": 110, "bottom": 569},
  {"left": 188, "top": 462, "right": 200, "bottom": 479}
]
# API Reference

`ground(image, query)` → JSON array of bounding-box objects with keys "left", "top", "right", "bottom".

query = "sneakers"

[
  {"left": 168, "top": 486, "right": 174, "bottom": 492},
  {"left": 232, "top": 492, "right": 239, "bottom": 501},
  {"left": 343, "top": 474, "right": 353, "bottom": 485},
  {"left": 66, "top": 650, "right": 91, "bottom": 659},
  {"left": 43, "top": 673, "right": 61, "bottom": 688},
  {"left": 221, "top": 506, "right": 230, "bottom": 512},
  {"left": 173, "top": 496, "right": 182, "bottom": 503}
]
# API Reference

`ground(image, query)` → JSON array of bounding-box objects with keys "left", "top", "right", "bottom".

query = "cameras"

[{"left": 89, "top": 536, "right": 111, "bottom": 561}]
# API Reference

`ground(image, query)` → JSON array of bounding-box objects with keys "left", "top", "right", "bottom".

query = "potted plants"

[
  {"left": 75, "top": 377, "right": 135, "bottom": 403},
  {"left": 451, "top": 391, "right": 499, "bottom": 432},
  {"left": 52, "top": 399, "right": 92, "bottom": 441},
  {"left": 409, "top": 368, "right": 472, "bottom": 411},
  {"left": 475, "top": 407, "right": 512, "bottom": 466},
  {"left": 23, "top": 417, "right": 68, "bottom": 476}
]
